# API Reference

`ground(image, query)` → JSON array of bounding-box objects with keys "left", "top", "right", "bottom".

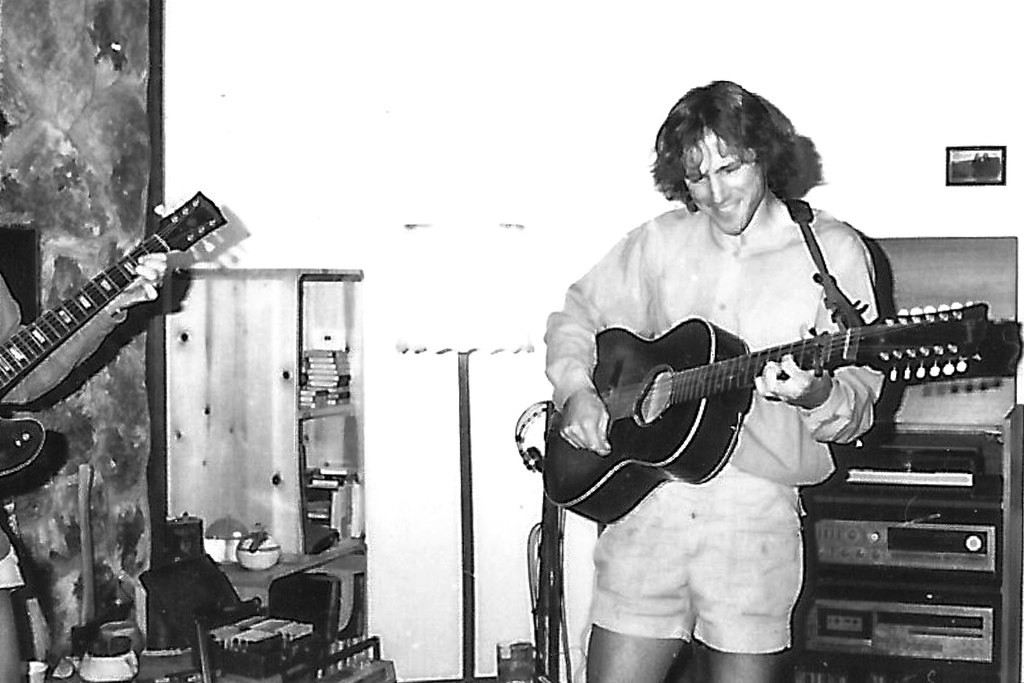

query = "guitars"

[
  {"left": 0, "top": 191, "right": 229, "bottom": 486},
  {"left": 542, "top": 301, "right": 990, "bottom": 525}
]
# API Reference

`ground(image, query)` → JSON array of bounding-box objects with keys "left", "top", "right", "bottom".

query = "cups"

[
  {"left": 205, "top": 535, "right": 240, "bottom": 564},
  {"left": 80, "top": 621, "right": 143, "bottom": 683},
  {"left": 28, "top": 661, "right": 48, "bottom": 682}
]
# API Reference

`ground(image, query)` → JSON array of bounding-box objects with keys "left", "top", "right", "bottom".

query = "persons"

[
  {"left": 544, "top": 79, "right": 883, "bottom": 683},
  {"left": 0, "top": 252, "right": 169, "bottom": 683}
]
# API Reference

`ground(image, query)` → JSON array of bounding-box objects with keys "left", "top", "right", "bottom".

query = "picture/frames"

[{"left": 945, "top": 145, "right": 1008, "bottom": 186}]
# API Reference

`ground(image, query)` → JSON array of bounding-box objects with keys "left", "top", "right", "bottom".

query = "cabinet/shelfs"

[
  {"left": 791, "top": 403, "right": 1024, "bottom": 683},
  {"left": 166, "top": 265, "right": 364, "bottom": 561}
]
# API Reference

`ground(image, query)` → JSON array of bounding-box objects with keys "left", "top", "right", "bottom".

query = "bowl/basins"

[{"left": 237, "top": 528, "right": 280, "bottom": 571}]
